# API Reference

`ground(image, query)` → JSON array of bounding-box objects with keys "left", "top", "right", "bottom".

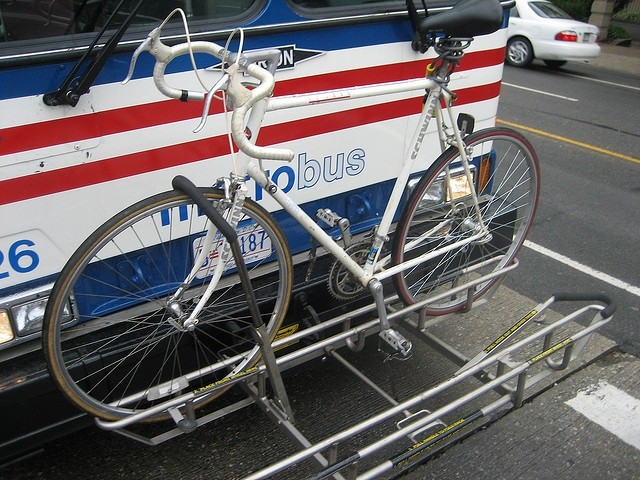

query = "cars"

[{"left": 504, "top": 0, "right": 601, "bottom": 67}]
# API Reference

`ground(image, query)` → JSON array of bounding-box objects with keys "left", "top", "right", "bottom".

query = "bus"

[{"left": 1, "top": 0, "right": 517, "bottom": 462}]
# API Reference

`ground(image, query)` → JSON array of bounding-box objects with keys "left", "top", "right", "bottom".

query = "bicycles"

[{"left": 42, "top": 0, "right": 543, "bottom": 425}]
